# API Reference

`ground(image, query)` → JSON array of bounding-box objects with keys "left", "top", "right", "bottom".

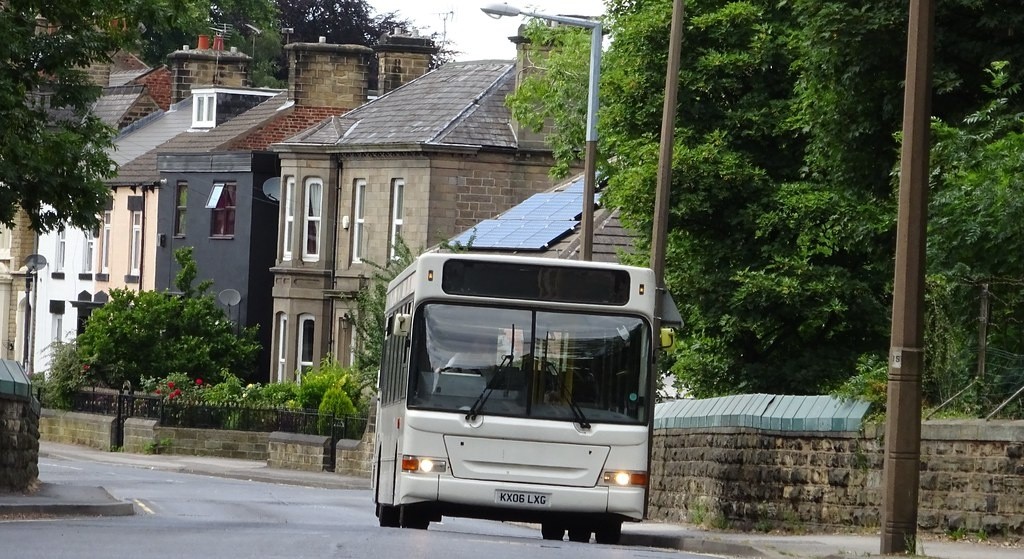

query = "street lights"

[{"left": 480, "top": 4, "right": 605, "bottom": 261}]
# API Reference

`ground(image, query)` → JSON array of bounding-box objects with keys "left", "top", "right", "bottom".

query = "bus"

[{"left": 370, "top": 251, "right": 655, "bottom": 546}]
